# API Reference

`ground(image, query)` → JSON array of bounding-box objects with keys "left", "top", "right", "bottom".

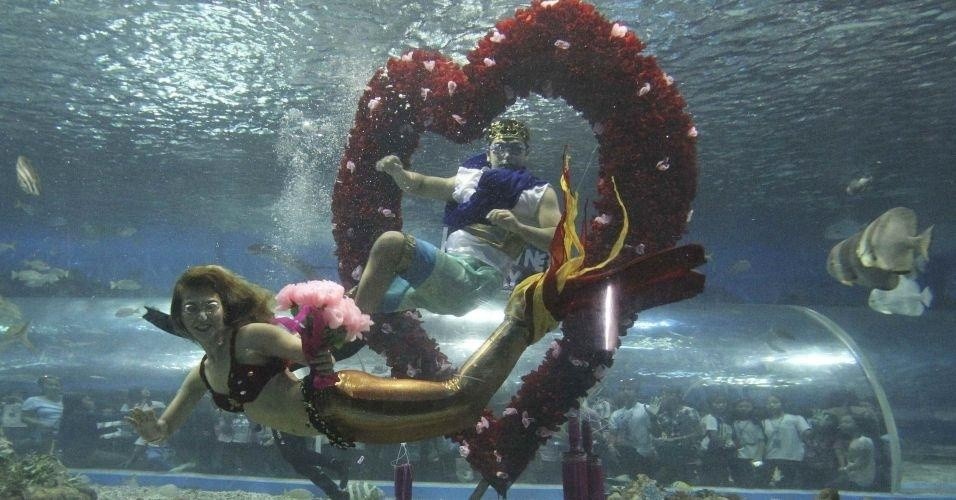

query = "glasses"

[
  {"left": 491, "top": 144, "right": 527, "bottom": 155},
  {"left": 184, "top": 301, "right": 218, "bottom": 313}
]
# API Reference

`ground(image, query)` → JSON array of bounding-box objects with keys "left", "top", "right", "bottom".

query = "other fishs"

[
  {"left": 248, "top": 241, "right": 327, "bottom": 283},
  {"left": 344, "top": 479, "right": 385, "bottom": 500},
  {"left": 825, "top": 204, "right": 936, "bottom": 318},
  {"left": 16, "top": 154, "right": 41, "bottom": 197},
  {"left": 844, "top": 175, "right": 873, "bottom": 195},
  {"left": 20, "top": 396, "right": 63, "bottom": 428},
  {"left": 729, "top": 260, "right": 754, "bottom": 277},
  {"left": 0, "top": 200, "right": 147, "bottom": 385}
]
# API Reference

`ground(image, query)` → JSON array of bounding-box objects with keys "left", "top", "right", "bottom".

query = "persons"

[
  {"left": 328, "top": 118, "right": 567, "bottom": 358},
  {"left": 1, "top": 376, "right": 891, "bottom": 492},
  {"left": 121, "top": 264, "right": 568, "bottom": 452}
]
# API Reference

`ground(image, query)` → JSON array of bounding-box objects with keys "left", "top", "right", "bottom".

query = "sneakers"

[{"left": 514, "top": 273, "right": 572, "bottom": 340}]
